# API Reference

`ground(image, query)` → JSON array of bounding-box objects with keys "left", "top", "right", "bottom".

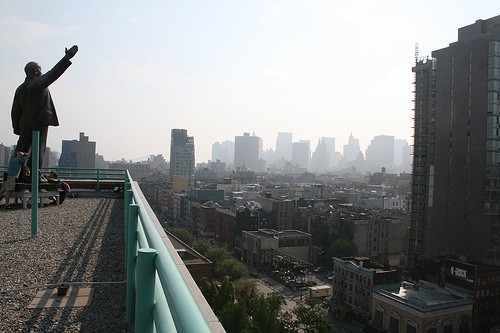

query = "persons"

[
  {"left": 10, "top": 45, "right": 78, "bottom": 178},
  {"left": 0, "top": 151, "right": 58, "bottom": 211}
]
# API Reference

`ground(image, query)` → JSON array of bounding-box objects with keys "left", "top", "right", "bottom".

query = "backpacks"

[{"left": 61, "top": 181, "right": 70, "bottom": 192}]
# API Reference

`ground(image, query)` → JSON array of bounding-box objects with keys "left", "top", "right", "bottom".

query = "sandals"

[{"left": 5, "top": 204, "right": 14, "bottom": 210}]
